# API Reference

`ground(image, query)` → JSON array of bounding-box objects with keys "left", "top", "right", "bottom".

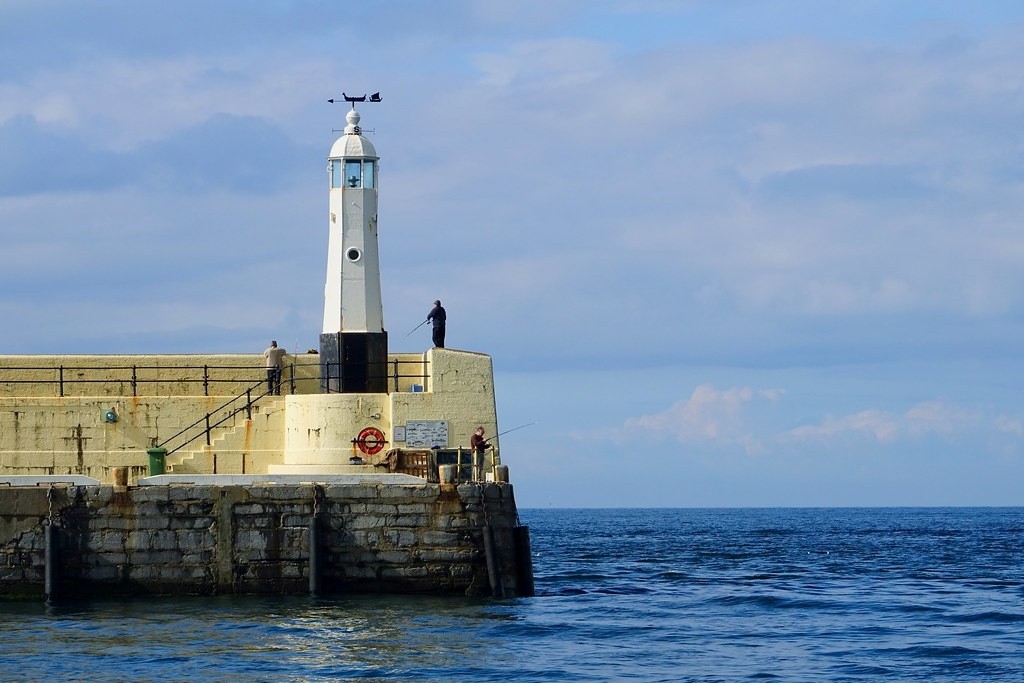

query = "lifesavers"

[{"left": 358, "top": 429, "right": 384, "bottom": 454}]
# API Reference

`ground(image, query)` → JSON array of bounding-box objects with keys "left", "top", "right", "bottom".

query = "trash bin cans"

[{"left": 147, "top": 448, "right": 166, "bottom": 477}]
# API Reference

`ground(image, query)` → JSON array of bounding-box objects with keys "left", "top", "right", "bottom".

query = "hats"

[{"left": 433, "top": 300, "right": 441, "bottom": 305}]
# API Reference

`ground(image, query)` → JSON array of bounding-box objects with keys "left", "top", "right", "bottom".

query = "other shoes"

[
  {"left": 275, "top": 393, "right": 281, "bottom": 396},
  {"left": 266, "top": 392, "right": 273, "bottom": 396},
  {"left": 477, "top": 480, "right": 484, "bottom": 483}
]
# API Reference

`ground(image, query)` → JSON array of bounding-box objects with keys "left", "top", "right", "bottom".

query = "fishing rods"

[
  {"left": 486, "top": 422, "right": 535, "bottom": 441},
  {"left": 407, "top": 319, "right": 431, "bottom": 335},
  {"left": 292, "top": 338, "right": 298, "bottom": 394}
]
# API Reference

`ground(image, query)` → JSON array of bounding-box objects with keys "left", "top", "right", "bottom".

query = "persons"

[
  {"left": 427, "top": 299, "right": 447, "bottom": 348},
  {"left": 470, "top": 427, "right": 492, "bottom": 484},
  {"left": 264, "top": 340, "right": 286, "bottom": 395}
]
